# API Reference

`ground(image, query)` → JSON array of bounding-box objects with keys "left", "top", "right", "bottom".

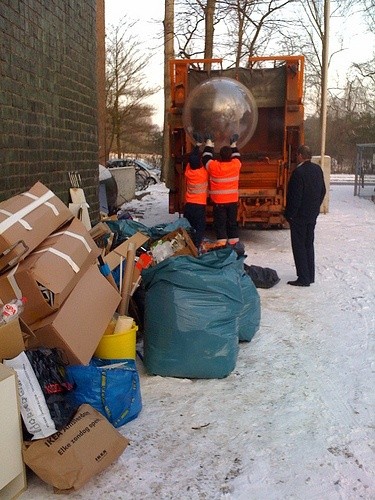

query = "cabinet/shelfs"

[{"left": 0, "top": 365, "right": 28, "bottom": 500}]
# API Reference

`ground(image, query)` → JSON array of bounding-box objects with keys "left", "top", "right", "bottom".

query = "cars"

[{"left": 110, "top": 158, "right": 161, "bottom": 185}]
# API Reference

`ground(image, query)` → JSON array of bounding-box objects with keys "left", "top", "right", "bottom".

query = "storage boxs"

[
  {"left": 0, "top": 216, "right": 101, "bottom": 326},
  {"left": 149, "top": 226, "right": 199, "bottom": 269},
  {"left": 22, "top": 264, "right": 125, "bottom": 368},
  {"left": 0, "top": 181, "right": 75, "bottom": 276},
  {"left": 0, "top": 311, "right": 37, "bottom": 365},
  {"left": 103, "top": 232, "right": 148, "bottom": 290}
]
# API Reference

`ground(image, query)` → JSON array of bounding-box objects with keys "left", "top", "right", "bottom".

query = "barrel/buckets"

[{"left": 93, "top": 320, "right": 138, "bottom": 360}]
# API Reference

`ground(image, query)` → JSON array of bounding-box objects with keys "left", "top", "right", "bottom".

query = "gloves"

[
  {"left": 193, "top": 134, "right": 201, "bottom": 141},
  {"left": 231, "top": 134, "right": 239, "bottom": 143},
  {"left": 203, "top": 133, "right": 216, "bottom": 141}
]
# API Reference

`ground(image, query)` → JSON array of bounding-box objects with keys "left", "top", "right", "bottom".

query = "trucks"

[{"left": 167, "top": 54, "right": 306, "bottom": 230}]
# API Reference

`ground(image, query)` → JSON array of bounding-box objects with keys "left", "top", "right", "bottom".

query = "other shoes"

[{"left": 287, "top": 281, "right": 310, "bottom": 286}]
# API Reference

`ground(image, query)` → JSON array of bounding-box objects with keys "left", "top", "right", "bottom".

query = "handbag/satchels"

[
  {"left": 3, "top": 347, "right": 78, "bottom": 441},
  {"left": 67, "top": 358, "right": 142, "bottom": 429},
  {"left": 22, "top": 404, "right": 131, "bottom": 494}
]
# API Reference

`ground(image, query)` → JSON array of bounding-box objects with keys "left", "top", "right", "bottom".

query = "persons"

[
  {"left": 282, "top": 145, "right": 327, "bottom": 287},
  {"left": 183, "top": 131, "right": 216, "bottom": 246},
  {"left": 203, "top": 128, "right": 242, "bottom": 245}
]
